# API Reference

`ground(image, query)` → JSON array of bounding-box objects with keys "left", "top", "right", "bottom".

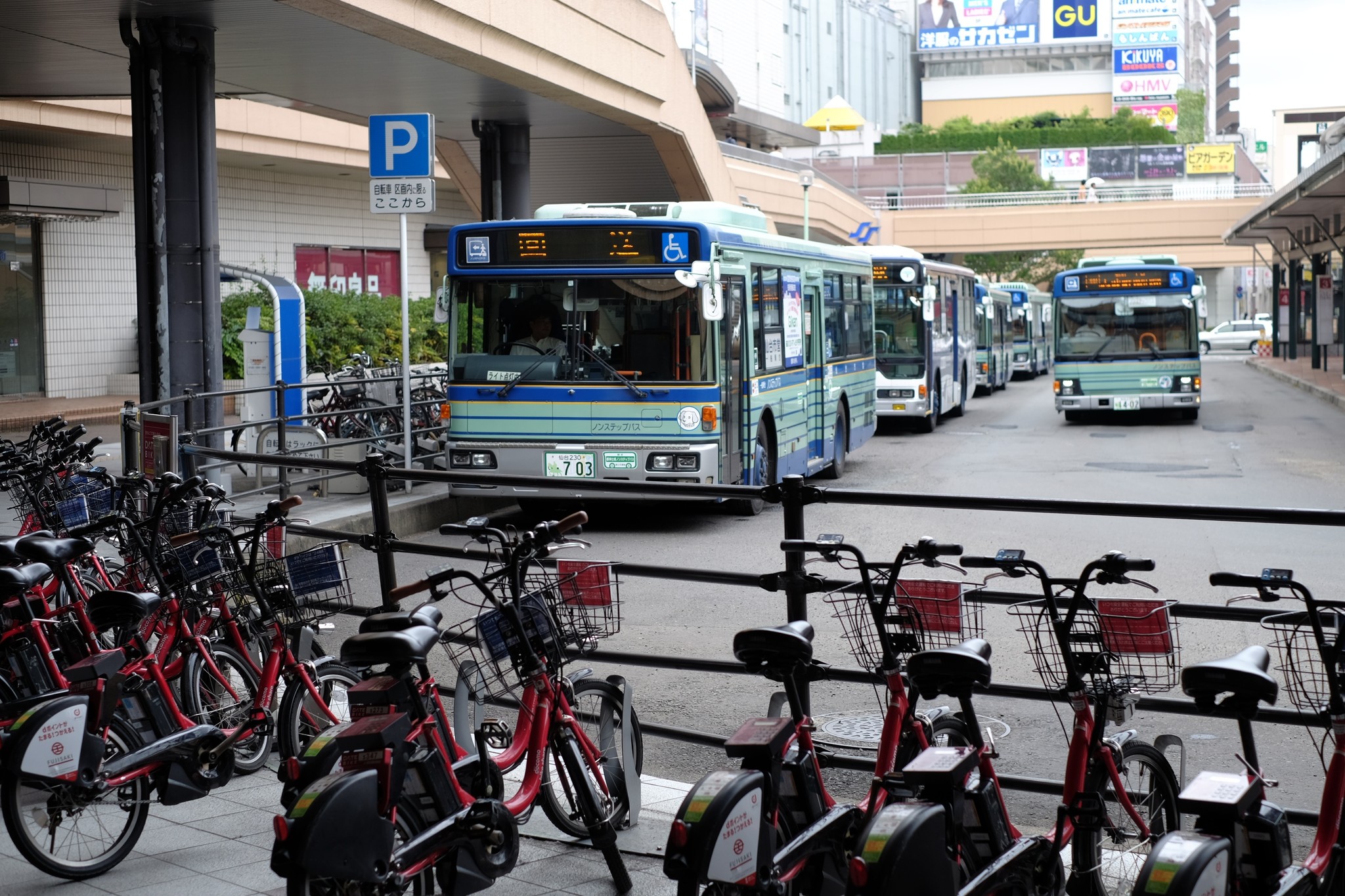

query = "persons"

[
  {"left": 510, "top": 312, "right": 569, "bottom": 363},
  {"left": 1075, "top": 310, "right": 1106, "bottom": 338}
]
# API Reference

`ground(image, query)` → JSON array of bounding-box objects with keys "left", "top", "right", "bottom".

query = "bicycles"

[
  {"left": 271, "top": 509, "right": 644, "bottom": 896},
  {"left": 1130, "top": 566, "right": 1345, "bottom": 896},
  {"left": 0, "top": 416, "right": 365, "bottom": 879},
  {"left": 850, "top": 548, "right": 1182, "bottom": 896},
  {"left": 231, "top": 351, "right": 449, "bottom": 476},
  {"left": 663, "top": 532, "right": 989, "bottom": 896}
]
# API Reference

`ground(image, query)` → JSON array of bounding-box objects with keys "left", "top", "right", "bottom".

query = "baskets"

[
  {"left": 1006, "top": 593, "right": 1181, "bottom": 694},
  {"left": 408, "top": 360, "right": 432, "bottom": 389},
  {"left": 2, "top": 426, "right": 354, "bottom": 625},
  {"left": 822, "top": 576, "right": 986, "bottom": 671},
  {"left": 336, "top": 369, "right": 363, "bottom": 391},
  {"left": 1259, "top": 609, "right": 1345, "bottom": 707},
  {"left": 364, "top": 378, "right": 396, "bottom": 407},
  {"left": 481, "top": 558, "right": 627, "bottom": 640},
  {"left": 371, "top": 365, "right": 402, "bottom": 386},
  {"left": 435, "top": 572, "right": 597, "bottom": 706}
]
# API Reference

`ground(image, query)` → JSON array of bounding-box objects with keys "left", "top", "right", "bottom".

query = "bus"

[
  {"left": 731, "top": 272, "right": 872, "bottom": 369},
  {"left": 847, "top": 245, "right": 976, "bottom": 432},
  {"left": 888, "top": 284, "right": 1014, "bottom": 396},
  {"left": 433, "top": 201, "right": 878, "bottom": 516},
  {"left": 991, "top": 283, "right": 1053, "bottom": 379},
  {"left": 1045, "top": 254, "right": 1208, "bottom": 422}
]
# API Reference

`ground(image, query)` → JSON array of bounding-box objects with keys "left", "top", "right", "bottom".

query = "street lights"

[{"left": 798, "top": 170, "right": 814, "bottom": 240}]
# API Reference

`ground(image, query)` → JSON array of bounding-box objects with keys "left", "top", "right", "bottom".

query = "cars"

[
  {"left": 818, "top": 150, "right": 838, "bottom": 156},
  {"left": 1198, "top": 320, "right": 1280, "bottom": 355}
]
